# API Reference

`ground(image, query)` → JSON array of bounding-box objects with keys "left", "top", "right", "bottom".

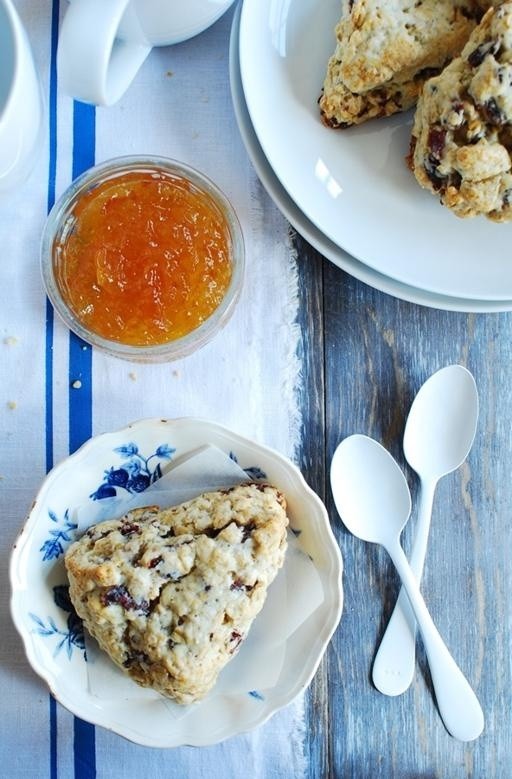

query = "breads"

[
  {"left": 63, "top": 484, "right": 288, "bottom": 701},
  {"left": 318, "top": 0, "right": 497, "bottom": 123},
  {"left": 405, "top": 0, "right": 512, "bottom": 224}
]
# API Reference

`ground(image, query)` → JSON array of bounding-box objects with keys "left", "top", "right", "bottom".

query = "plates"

[
  {"left": 7, "top": 416, "right": 349, "bottom": 751},
  {"left": 227, "top": 2, "right": 511, "bottom": 316}
]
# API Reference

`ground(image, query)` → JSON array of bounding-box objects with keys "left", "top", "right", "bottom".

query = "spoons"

[
  {"left": 329, "top": 434, "right": 486, "bottom": 744},
  {"left": 368, "top": 365, "right": 479, "bottom": 697}
]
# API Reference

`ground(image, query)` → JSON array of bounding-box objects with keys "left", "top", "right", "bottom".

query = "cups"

[
  {"left": 41, "top": 155, "right": 245, "bottom": 364},
  {"left": 55, "top": 0, "right": 235, "bottom": 111},
  {"left": 1, "top": 2, "right": 40, "bottom": 178}
]
